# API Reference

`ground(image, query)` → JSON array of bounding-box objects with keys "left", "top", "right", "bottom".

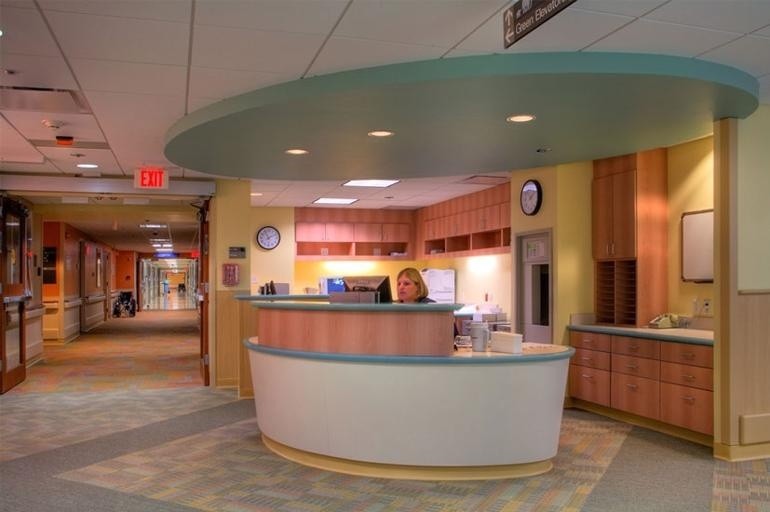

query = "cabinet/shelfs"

[
  {"left": 296, "top": 223, "right": 354, "bottom": 261},
  {"left": 425, "top": 202, "right": 511, "bottom": 261},
  {"left": 354, "top": 223, "right": 409, "bottom": 260},
  {"left": 663, "top": 341, "right": 713, "bottom": 437},
  {"left": 592, "top": 147, "right": 669, "bottom": 327},
  {"left": 569, "top": 330, "right": 611, "bottom": 407},
  {"left": 612, "top": 334, "right": 661, "bottom": 422}
]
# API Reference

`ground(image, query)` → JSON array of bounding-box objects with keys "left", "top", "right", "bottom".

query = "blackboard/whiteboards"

[{"left": 680, "top": 209, "right": 715, "bottom": 281}]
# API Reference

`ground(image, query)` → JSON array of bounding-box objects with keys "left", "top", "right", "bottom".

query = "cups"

[{"left": 471, "top": 321, "right": 489, "bottom": 352}]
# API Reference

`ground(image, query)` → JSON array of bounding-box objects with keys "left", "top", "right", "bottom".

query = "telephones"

[{"left": 649, "top": 313, "right": 689, "bottom": 329}]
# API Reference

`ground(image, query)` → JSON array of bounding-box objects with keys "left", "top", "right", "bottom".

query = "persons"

[{"left": 396, "top": 268, "right": 460, "bottom": 341}]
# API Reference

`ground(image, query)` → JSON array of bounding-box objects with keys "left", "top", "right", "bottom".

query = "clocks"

[
  {"left": 257, "top": 225, "right": 281, "bottom": 250},
  {"left": 518, "top": 179, "right": 542, "bottom": 215}
]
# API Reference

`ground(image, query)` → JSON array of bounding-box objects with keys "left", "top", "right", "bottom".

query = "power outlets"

[{"left": 704, "top": 300, "right": 711, "bottom": 309}]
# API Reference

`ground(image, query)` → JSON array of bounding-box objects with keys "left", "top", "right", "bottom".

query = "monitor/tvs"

[{"left": 342, "top": 276, "right": 392, "bottom": 303}]
